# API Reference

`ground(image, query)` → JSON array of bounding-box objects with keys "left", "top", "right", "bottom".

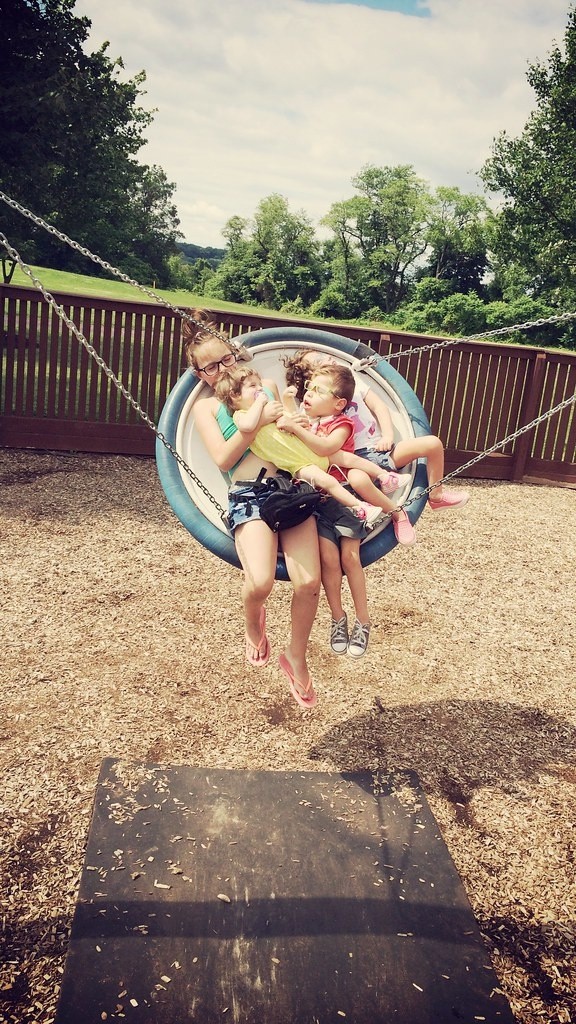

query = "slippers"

[
  {"left": 245, "top": 607, "right": 269, "bottom": 668},
  {"left": 278, "top": 655, "right": 317, "bottom": 709}
]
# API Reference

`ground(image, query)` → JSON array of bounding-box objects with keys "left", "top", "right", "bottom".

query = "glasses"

[
  {"left": 196, "top": 352, "right": 236, "bottom": 376},
  {"left": 304, "top": 380, "right": 340, "bottom": 400}
]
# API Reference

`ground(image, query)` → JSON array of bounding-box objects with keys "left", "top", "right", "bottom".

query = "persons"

[
  {"left": 179, "top": 304, "right": 323, "bottom": 709},
  {"left": 276, "top": 362, "right": 372, "bottom": 659},
  {"left": 214, "top": 365, "right": 413, "bottom": 524},
  {"left": 278, "top": 345, "right": 473, "bottom": 547}
]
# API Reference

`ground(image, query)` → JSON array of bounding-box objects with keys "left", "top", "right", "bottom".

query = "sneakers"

[
  {"left": 428, "top": 492, "right": 471, "bottom": 511},
  {"left": 347, "top": 617, "right": 371, "bottom": 659},
  {"left": 330, "top": 611, "right": 349, "bottom": 656},
  {"left": 392, "top": 507, "right": 416, "bottom": 547}
]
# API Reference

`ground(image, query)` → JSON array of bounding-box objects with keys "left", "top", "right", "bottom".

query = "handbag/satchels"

[{"left": 260, "top": 477, "right": 321, "bottom": 533}]
